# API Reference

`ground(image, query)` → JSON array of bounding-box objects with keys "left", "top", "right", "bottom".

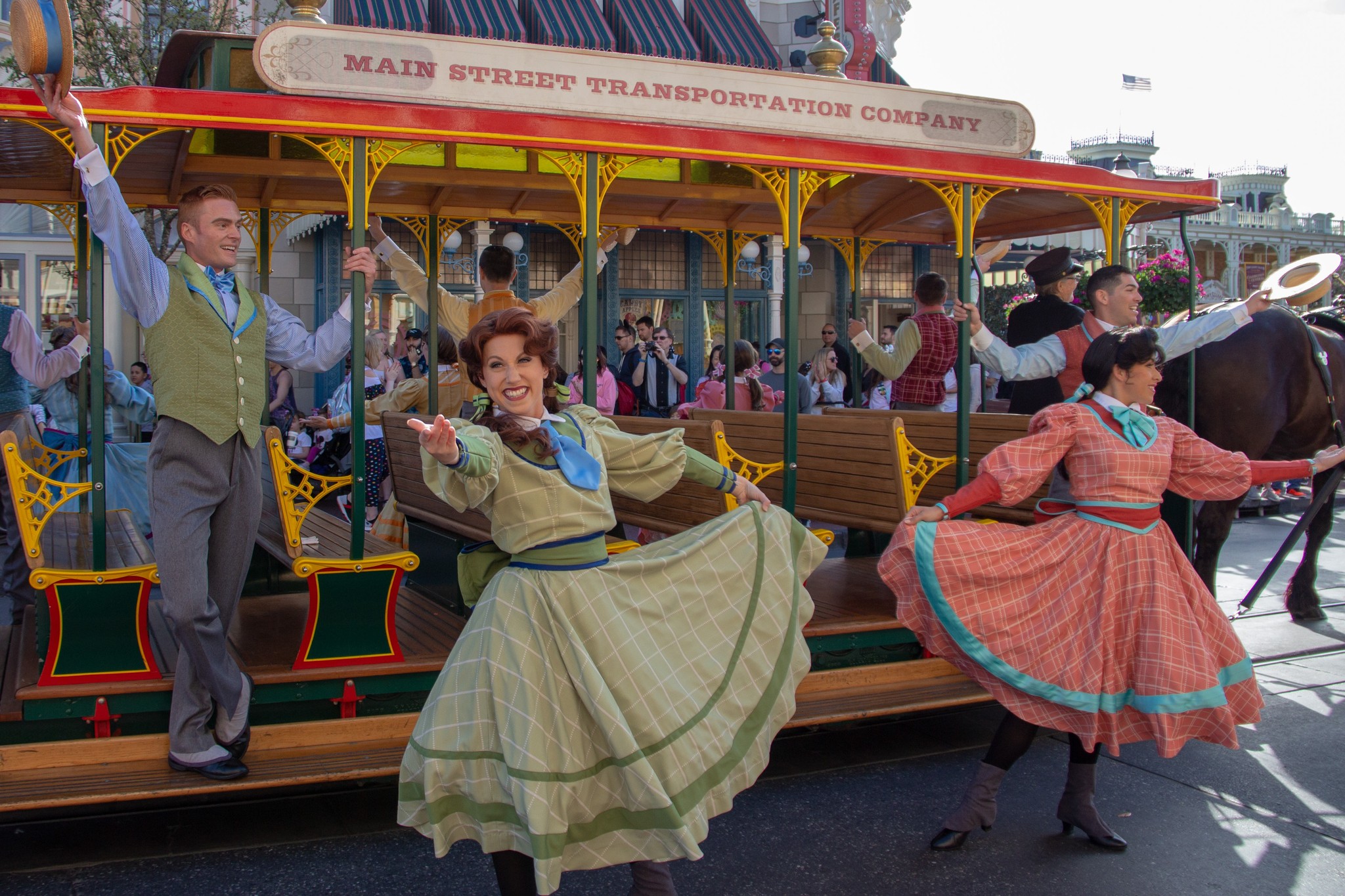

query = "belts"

[{"left": 648, "top": 405, "right": 671, "bottom": 413}]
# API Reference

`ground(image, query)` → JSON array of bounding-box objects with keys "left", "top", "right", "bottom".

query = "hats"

[
  {"left": 765, "top": 338, "right": 785, "bottom": 349},
  {"left": 1025, "top": 247, "right": 1084, "bottom": 286},
  {"left": 404, "top": 328, "right": 422, "bottom": 340}
]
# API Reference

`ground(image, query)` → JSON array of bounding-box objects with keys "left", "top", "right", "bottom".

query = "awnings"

[{"left": 334, "top": 0, "right": 783, "bottom": 71}]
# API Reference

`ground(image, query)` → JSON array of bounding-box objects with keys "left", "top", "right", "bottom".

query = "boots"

[
  {"left": 628, "top": 860, "right": 678, "bottom": 896},
  {"left": 491, "top": 850, "right": 537, "bottom": 896}
]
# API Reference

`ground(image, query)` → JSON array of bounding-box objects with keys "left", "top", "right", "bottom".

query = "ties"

[{"left": 539, "top": 419, "right": 600, "bottom": 490}]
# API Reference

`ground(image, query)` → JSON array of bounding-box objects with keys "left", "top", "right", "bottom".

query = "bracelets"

[
  {"left": 665, "top": 361, "right": 670, "bottom": 366},
  {"left": 639, "top": 358, "right": 646, "bottom": 362},
  {"left": 821, "top": 378, "right": 827, "bottom": 382}
]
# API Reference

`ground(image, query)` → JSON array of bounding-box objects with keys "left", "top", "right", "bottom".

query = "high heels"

[
  {"left": 930, "top": 823, "right": 993, "bottom": 852},
  {"left": 1055, "top": 809, "right": 1128, "bottom": 849}
]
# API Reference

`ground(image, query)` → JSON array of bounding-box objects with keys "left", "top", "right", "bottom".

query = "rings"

[
  {"left": 640, "top": 346, "right": 642, "bottom": 349},
  {"left": 396, "top": 370, "right": 398, "bottom": 373}
]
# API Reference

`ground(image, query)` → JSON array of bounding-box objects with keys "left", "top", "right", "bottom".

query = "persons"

[
  {"left": 563, "top": 314, "right": 689, "bottom": 421},
  {"left": 395, "top": 307, "right": 829, "bottom": 896},
  {"left": 757, "top": 339, "right": 812, "bottom": 414},
  {"left": 368, "top": 214, "right": 621, "bottom": 420},
  {"left": 751, "top": 342, "right": 770, "bottom": 375},
  {"left": 677, "top": 338, "right": 784, "bottom": 420},
  {"left": 1248, "top": 477, "right": 1309, "bottom": 501},
  {"left": 0, "top": 266, "right": 462, "bottom": 626},
  {"left": 848, "top": 254, "right": 990, "bottom": 412},
  {"left": 23, "top": 74, "right": 379, "bottom": 779},
  {"left": 804, "top": 322, "right": 899, "bottom": 414},
  {"left": 1007, "top": 247, "right": 1086, "bottom": 416},
  {"left": 696, "top": 344, "right": 724, "bottom": 388},
  {"left": 874, "top": 322, "right": 1345, "bottom": 855},
  {"left": 953, "top": 264, "right": 1272, "bottom": 506},
  {"left": 943, "top": 346, "right": 1015, "bottom": 413}
]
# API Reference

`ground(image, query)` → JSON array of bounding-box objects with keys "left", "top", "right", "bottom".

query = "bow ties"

[
  {"left": 1110, "top": 405, "right": 1155, "bottom": 448},
  {"left": 204, "top": 266, "right": 235, "bottom": 293}
]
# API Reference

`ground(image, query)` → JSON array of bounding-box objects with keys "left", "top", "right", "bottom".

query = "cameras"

[{"left": 645, "top": 341, "right": 656, "bottom": 351}]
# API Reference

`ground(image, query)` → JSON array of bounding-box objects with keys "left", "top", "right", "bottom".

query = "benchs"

[{"left": 0, "top": 406, "right": 1045, "bottom": 689}]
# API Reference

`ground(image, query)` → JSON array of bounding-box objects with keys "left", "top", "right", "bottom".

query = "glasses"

[
  {"left": 652, "top": 335, "right": 668, "bottom": 341},
  {"left": 615, "top": 335, "right": 630, "bottom": 340},
  {"left": 766, "top": 349, "right": 784, "bottom": 355},
  {"left": 578, "top": 354, "right": 584, "bottom": 361},
  {"left": 1067, "top": 274, "right": 1081, "bottom": 282},
  {"left": 822, "top": 330, "right": 836, "bottom": 335},
  {"left": 827, "top": 356, "right": 838, "bottom": 362}
]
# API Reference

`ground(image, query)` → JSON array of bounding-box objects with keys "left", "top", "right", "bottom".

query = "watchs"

[{"left": 411, "top": 361, "right": 419, "bottom": 368}]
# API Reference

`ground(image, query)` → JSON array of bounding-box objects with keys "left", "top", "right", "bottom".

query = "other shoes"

[
  {"left": 297, "top": 463, "right": 310, "bottom": 472},
  {"left": 215, "top": 672, "right": 254, "bottom": 760},
  {"left": 168, "top": 750, "right": 249, "bottom": 780},
  {"left": 336, "top": 495, "right": 352, "bottom": 524},
  {"left": 364, "top": 516, "right": 379, "bottom": 531},
  {"left": 1245, "top": 485, "right": 1307, "bottom": 502}
]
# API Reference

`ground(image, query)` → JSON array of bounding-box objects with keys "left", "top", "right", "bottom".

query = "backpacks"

[{"left": 327, "top": 371, "right": 352, "bottom": 434}]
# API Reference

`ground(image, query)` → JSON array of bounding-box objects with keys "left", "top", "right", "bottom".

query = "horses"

[{"left": 1147, "top": 301, "right": 1345, "bottom": 622}]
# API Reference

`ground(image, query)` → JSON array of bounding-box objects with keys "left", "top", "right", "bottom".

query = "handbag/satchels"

[{"left": 616, "top": 380, "right": 636, "bottom": 416}]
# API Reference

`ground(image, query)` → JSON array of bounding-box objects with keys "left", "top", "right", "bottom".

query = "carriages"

[{"left": 0, "top": 28, "right": 1344, "bottom": 826}]
